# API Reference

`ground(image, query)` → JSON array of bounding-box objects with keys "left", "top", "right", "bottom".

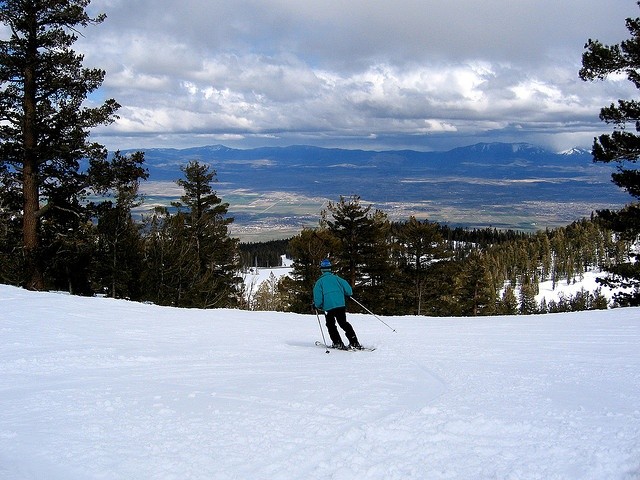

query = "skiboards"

[{"left": 316, "top": 340, "right": 377, "bottom": 353}]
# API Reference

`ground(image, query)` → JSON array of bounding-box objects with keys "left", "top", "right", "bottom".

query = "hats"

[{"left": 320, "top": 258, "right": 332, "bottom": 272}]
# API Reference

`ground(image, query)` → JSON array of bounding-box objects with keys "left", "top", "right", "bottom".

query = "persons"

[{"left": 313, "top": 259, "right": 362, "bottom": 350}]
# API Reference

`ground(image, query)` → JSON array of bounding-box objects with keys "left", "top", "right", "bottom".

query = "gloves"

[{"left": 311, "top": 304, "right": 317, "bottom": 312}]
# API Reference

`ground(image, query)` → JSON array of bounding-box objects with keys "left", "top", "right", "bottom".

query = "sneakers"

[
  {"left": 348, "top": 342, "right": 361, "bottom": 350},
  {"left": 332, "top": 341, "right": 344, "bottom": 349}
]
